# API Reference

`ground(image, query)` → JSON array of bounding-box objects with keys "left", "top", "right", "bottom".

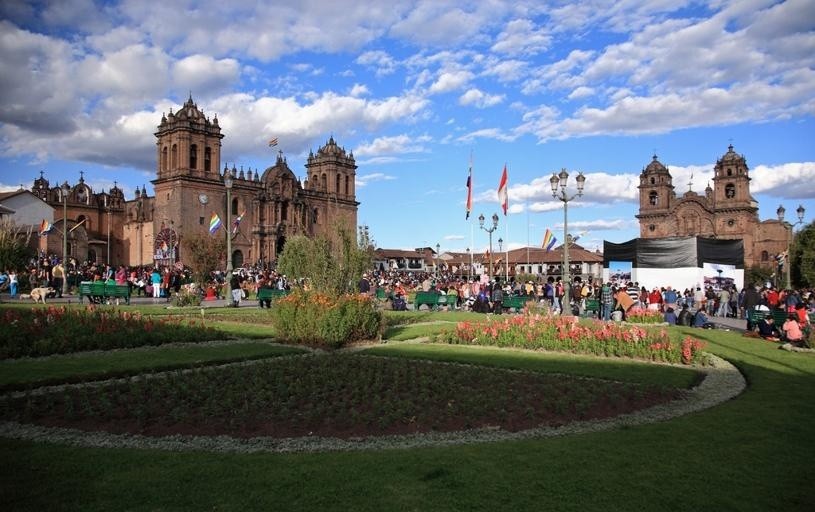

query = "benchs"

[
  {"left": 584, "top": 300, "right": 615, "bottom": 316},
  {"left": 747, "top": 308, "right": 808, "bottom": 340},
  {"left": 498, "top": 296, "right": 534, "bottom": 314},
  {"left": 80, "top": 282, "right": 130, "bottom": 306},
  {"left": 258, "top": 286, "right": 294, "bottom": 310},
  {"left": 376, "top": 287, "right": 396, "bottom": 302},
  {"left": 415, "top": 292, "right": 461, "bottom": 313}
]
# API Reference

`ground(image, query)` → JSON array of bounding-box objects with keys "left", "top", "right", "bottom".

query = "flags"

[
  {"left": 497, "top": 162, "right": 509, "bottom": 216},
  {"left": 542, "top": 229, "right": 557, "bottom": 253},
  {"left": 231, "top": 210, "right": 248, "bottom": 233},
  {"left": 208, "top": 212, "right": 223, "bottom": 236},
  {"left": 466, "top": 165, "right": 472, "bottom": 221},
  {"left": 39, "top": 219, "right": 58, "bottom": 236}
]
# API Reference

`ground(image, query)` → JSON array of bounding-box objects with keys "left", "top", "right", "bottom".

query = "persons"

[
  {"left": 568, "top": 275, "right": 739, "bottom": 330},
  {"left": 204, "top": 262, "right": 310, "bottom": 308},
  {"left": 2, "top": 256, "right": 193, "bottom": 299},
  {"left": 360, "top": 265, "right": 564, "bottom": 315},
  {"left": 737, "top": 282, "right": 813, "bottom": 348}
]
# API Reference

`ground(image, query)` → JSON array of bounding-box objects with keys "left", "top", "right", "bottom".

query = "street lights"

[
  {"left": 776, "top": 202, "right": 808, "bottom": 288},
  {"left": 478, "top": 211, "right": 499, "bottom": 282},
  {"left": 466, "top": 247, "right": 470, "bottom": 281},
  {"left": 223, "top": 167, "right": 236, "bottom": 305},
  {"left": 549, "top": 167, "right": 586, "bottom": 316},
  {"left": 59, "top": 179, "right": 70, "bottom": 296},
  {"left": 167, "top": 219, "right": 176, "bottom": 264},
  {"left": 435, "top": 241, "right": 441, "bottom": 277},
  {"left": 498, "top": 236, "right": 503, "bottom": 276}
]
